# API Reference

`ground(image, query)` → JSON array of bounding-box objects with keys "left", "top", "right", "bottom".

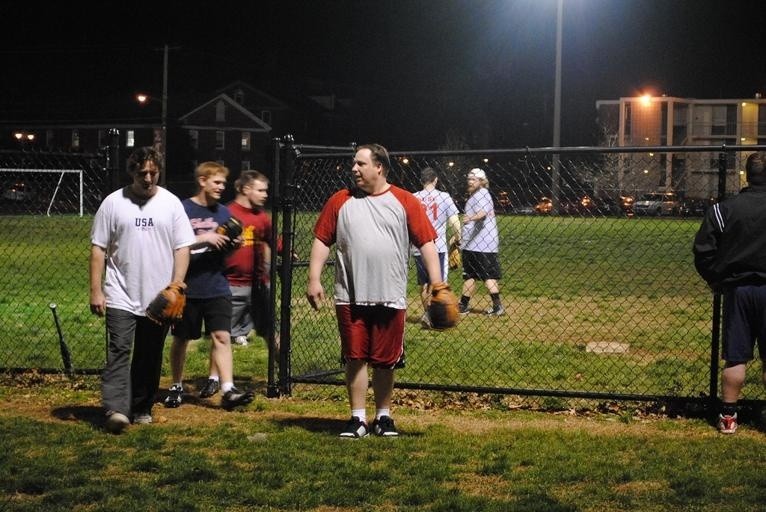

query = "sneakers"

[
  {"left": 485, "top": 302, "right": 505, "bottom": 316},
  {"left": 165, "top": 382, "right": 184, "bottom": 408},
  {"left": 134, "top": 414, "right": 152, "bottom": 423},
  {"left": 200, "top": 379, "right": 219, "bottom": 398},
  {"left": 371, "top": 416, "right": 400, "bottom": 437},
  {"left": 454, "top": 300, "right": 470, "bottom": 315},
  {"left": 340, "top": 415, "right": 369, "bottom": 441},
  {"left": 717, "top": 412, "right": 738, "bottom": 434},
  {"left": 421, "top": 310, "right": 434, "bottom": 329},
  {"left": 221, "top": 387, "right": 255, "bottom": 410},
  {"left": 106, "top": 410, "right": 129, "bottom": 431}
]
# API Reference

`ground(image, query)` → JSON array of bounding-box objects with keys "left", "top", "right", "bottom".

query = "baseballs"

[{"left": 235, "top": 334, "right": 249, "bottom": 343}]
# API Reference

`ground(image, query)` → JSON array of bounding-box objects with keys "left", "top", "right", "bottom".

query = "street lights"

[
  {"left": 11, "top": 125, "right": 38, "bottom": 181},
  {"left": 132, "top": 91, "right": 168, "bottom": 187}
]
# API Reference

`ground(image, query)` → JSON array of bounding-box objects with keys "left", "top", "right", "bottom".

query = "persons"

[
  {"left": 457, "top": 168, "right": 505, "bottom": 317},
  {"left": 166, "top": 160, "right": 254, "bottom": 412},
  {"left": 88, "top": 146, "right": 197, "bottom": 428},
  {"left": 198, "top": 168, "right": 298, "bottom": 399},
  {"left": 411, "top": 168, "right": 461, "bottom": 329},
  {"left": 692, "top": 152, "right": 766, "bottom": 433},
  {"left": 306, "top": 144, "right": 446, "bottom": 440}
]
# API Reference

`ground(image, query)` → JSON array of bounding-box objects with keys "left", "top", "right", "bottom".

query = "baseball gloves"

[
  {"left": 146, "top": 282, "right": 188, "bottom": 325},
  {"left": 428, "top": 282, "right": 460, "bottom": 327},
  {"left": 216, "top": 216, "right": 242, "bottom": 237}
]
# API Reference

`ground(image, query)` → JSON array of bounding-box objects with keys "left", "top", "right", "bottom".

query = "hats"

[{"left": 467, "top": 167, "right": 487, "bottom": 181}]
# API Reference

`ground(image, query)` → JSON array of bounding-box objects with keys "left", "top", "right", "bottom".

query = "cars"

[
  {"left": 493, "top": 184, "right": 714, "bottom": 218},
  {"left": 1, "top": 179, "right": 37, "bottom": 202}
]
